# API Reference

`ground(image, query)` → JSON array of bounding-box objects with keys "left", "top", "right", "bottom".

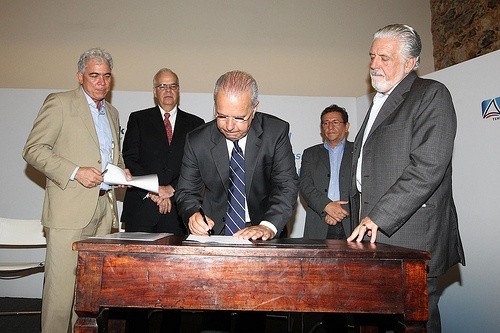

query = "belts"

[{"left": 99, "top": 189, "right": 111, "bottom": 196}]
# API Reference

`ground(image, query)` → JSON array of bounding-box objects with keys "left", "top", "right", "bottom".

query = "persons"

[
  {"left": 347, "top": 24, "right": 466, "bottom": 333},
  {"left": 299, "top": 104, "right": 354, "bottom": 240},
  {"left": 175, "top": 70, "right": 299, "bottom": 241},
  {"left": 120, "top": 68, "right": 205, "bottom": 233},
  {"left": 22, "top": 48, "right": 133, "bottom": 333}
]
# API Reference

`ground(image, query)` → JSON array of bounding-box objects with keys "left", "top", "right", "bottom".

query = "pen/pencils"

[
  {"left": 100, "top": 169, "right": 108, "bottom": 176},
  {"left": 197, "top": 205, "right": 211, "bottom": 236}
]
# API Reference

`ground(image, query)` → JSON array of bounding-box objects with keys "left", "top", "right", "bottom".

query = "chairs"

[{"left": 0, "top": 217, "right": 47, "bottom": 316}]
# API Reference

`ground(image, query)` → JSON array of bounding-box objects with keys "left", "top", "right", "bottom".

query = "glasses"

[
  {"left": 155, "top": 84, "right": 179, "bottom": 90},
  {"left": 322, "top": 120, "right": 347, "bottom": 127},
  {"left": 213, "top": 102, "right": 259, "bottom": 123}
]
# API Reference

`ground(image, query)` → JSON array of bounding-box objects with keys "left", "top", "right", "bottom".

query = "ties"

[
  {"left": 163, "top": 112, "right": 172, "bottom": 146},
  {"left": 224, "top": 139, "right": 246, "bottom": 236}
]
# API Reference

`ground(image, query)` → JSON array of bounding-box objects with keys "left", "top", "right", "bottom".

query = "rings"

[{"left": 362, "top": 224, "right": 368, "bottom": 231}]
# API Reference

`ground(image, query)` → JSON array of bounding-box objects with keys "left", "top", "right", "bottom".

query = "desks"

[{"left": 72, "top": 232, "right": 432, "bottom": 333}]
